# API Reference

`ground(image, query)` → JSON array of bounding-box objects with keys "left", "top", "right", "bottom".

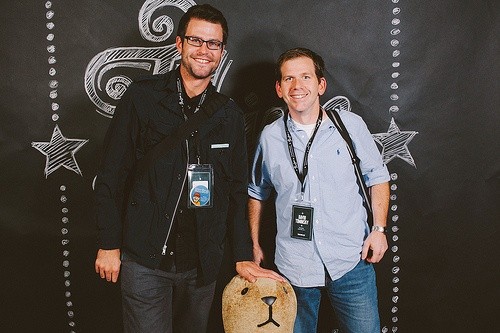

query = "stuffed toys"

[{"left": 222, "top": 268, "right": 298, "bottom": 333}]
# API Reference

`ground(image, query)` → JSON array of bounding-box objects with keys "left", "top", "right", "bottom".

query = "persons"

[
  {"left": 250, "top": 47, "right": 391, "bottom": 333},
  {"left": 94, "top": 5, "right": 248, "bottom": 333}
]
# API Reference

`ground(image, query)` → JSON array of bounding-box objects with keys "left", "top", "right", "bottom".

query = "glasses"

[{"left": 179, "top": 33, "right": 225, "bottom": 51}]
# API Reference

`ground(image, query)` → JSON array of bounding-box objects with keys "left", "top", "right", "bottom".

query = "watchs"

[{"left": 371, "top": 225, "right": 387, "bottom": 233}]
